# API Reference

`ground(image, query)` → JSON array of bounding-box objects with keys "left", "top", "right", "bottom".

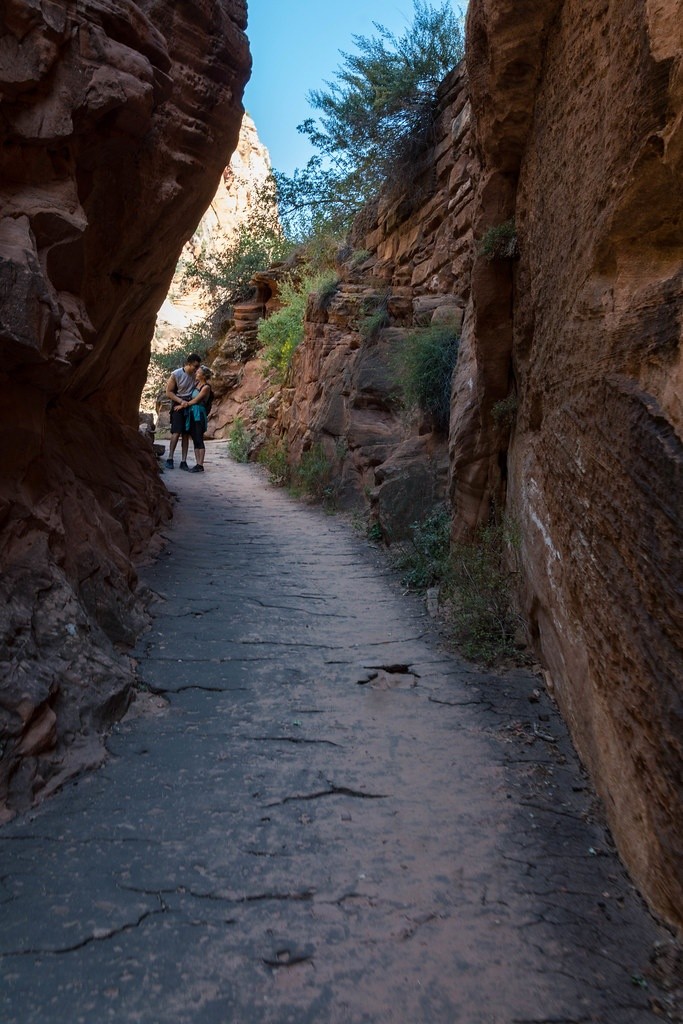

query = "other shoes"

[
  {"left": 165, "top": 459, "right": 174, "bottom": 469},
  {"left": 188, "top": 464, "right": 204, "bottom": 473},
  {"left": 180, "top": 462, "right": 189, "bottom": 471}
]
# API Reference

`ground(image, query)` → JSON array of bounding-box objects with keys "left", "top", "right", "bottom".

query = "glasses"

[{"left": 191, "top": 364, "right": 200, "bottom": 368}]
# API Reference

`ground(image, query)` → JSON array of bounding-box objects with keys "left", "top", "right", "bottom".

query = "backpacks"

[{"left": 194, "top": 381, "right": 214, "bottom": 416}]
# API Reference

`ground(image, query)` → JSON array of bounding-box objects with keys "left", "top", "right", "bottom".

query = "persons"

[
  {"left": 174, "top": 365, "right": 215, "bottom": 473},
  {"left": 165, "top": 354, "right": 202, "bottom": 471}
]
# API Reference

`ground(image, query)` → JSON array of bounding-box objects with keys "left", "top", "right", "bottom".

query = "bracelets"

[{"left": 179, "top": 400, "right": 183, "bottom": 404}]
